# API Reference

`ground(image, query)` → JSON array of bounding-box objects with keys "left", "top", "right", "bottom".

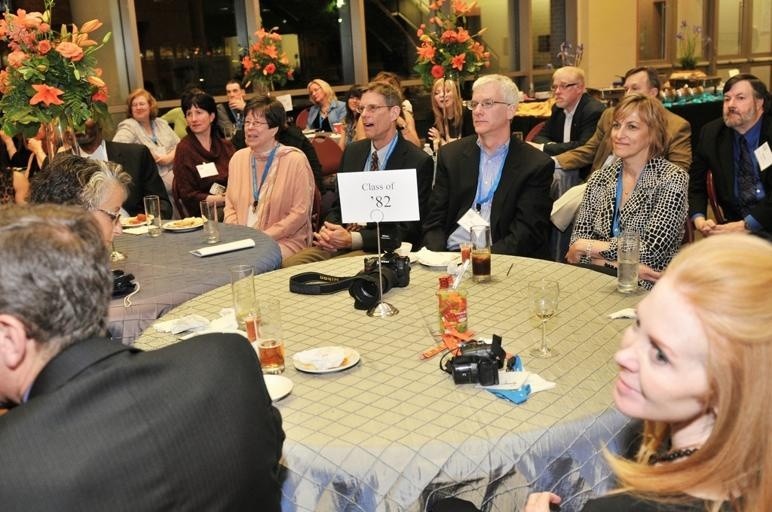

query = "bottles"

[{"left": 423, "top": 143, "right": 434, "bottom": 160}]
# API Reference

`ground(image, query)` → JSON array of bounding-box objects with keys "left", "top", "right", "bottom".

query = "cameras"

[
  {"left": 348, "top": 254, "right": 410, "bottom": 310},
  {"left": 449, "top": 358, "right": 499, "bottom": 385},
  {"left": 459, "top": 333, "right": 505, "bottom": 369}
]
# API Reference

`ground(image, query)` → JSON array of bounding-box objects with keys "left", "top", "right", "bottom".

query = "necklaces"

[{"left": 648, "top": 445, "right": 699, "bottom": 464}]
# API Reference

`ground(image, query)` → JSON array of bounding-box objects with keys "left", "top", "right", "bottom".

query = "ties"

[
  {"left": 738, "top": 136, "right": 759, "bottom": 219},
  {"left": 338, "top": 151, "right": 379, "bottom": 251}
]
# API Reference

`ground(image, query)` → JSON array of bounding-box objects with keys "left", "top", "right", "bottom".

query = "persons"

[
  {"left": 524, "top": 233, "right": 772, "bottom": 511},
  {"left": 1, "top": 203, "right": 286, "bottom": 511},
  {"left": 1, "top": 67, "right": 772, "bottom": 289}
]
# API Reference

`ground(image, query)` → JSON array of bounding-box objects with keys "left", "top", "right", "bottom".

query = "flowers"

[
  {"left": 411, "top": 1, "right": 492, "bottom": 84},
  {"left": 0, "top": 0, "right": 110, "bottom": 138},
  {"left": 675, "top": 19, "right": 712, "bottom": 70},
  {"left": 547, "top": 42, "right": 585, "bottom": 70},
  {"left": 236, "top": 28, "right": 294, "bottom": 86}
]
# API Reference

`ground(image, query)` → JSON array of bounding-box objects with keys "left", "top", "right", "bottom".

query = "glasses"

[
  {"left": 242, "top": 119, "right": 269, "bottom": 127},
  {"left": 355, "top": 104, "right": 394, "bottom": 113},
  {"left": 550, "top": 83, "right": 577, "bottom": 91},
  {"left": 98, "top": 208, "right": 121, "bottom": 226},
  {"left": 465, "top": 97, "right": 510, "bottom": 111}
]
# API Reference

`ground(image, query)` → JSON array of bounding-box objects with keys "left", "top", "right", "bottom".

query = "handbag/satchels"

[{"left": 550, "top": 182, "right": 589, "bottom": 233}]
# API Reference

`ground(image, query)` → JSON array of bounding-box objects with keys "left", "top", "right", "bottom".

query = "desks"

[{"left": 102, "top": 90, "right": 728, "bottom": 512}]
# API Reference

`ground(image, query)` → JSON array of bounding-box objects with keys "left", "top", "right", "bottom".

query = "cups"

[
  {"left": 459, "top": 243, "right": 472, "bottom": 265},
  {"left": 200, "top": 200, "right": 220, "bottom": 244},
  {"left": 247, "top": 296, "right": 286, "bottom": 376},
  {"left": 616, "top": 233, "right": 640, "bottom": 293},
  {"left": 230, "top": 262, "right": 259, "bottom": 330},
  {"left": 435, "top": 286, "right": 468, "bottom": 341},
  {"left": 143, "top": 195, "right": 162, "bottom": 239},
  {"left": 332, "top": 122, "right": 343, "bottom": 134},
  {"left": 471, "top": 226, "right": 491, "bottom": 284}
]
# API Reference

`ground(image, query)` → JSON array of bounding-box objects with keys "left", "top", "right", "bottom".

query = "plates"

[
  {"left": 420, "top": 250, "right": 463, "bottom": 268},
  {"left": 162, "top": 217, "right": 206, "bottom": 233},
  {"left": 119, "top": 214, "right": 155, "bottom": 228},
  {"left": 289, "top": 346, "right": 361, "bottom": 373},
  {"left": 259, "top": 372, "right": 294, "bottom": 403},
  {"left": 393, "top": 251, "right": 421, "bottom": 265}
]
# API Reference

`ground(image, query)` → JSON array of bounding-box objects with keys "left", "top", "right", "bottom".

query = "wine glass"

[{"left": 528, "top": 280, "right": 560, "bottom": 359}]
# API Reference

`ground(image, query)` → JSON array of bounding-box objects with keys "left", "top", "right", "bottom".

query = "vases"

[
  {"left": 44, "top": 118, "right": 80, "bottom": 164},
  {"left": 254, "top": 81, "right": 271, "bottom": 96},
  {"left": 447, "top": 74, "right": 463, "bottom": 99}
]
